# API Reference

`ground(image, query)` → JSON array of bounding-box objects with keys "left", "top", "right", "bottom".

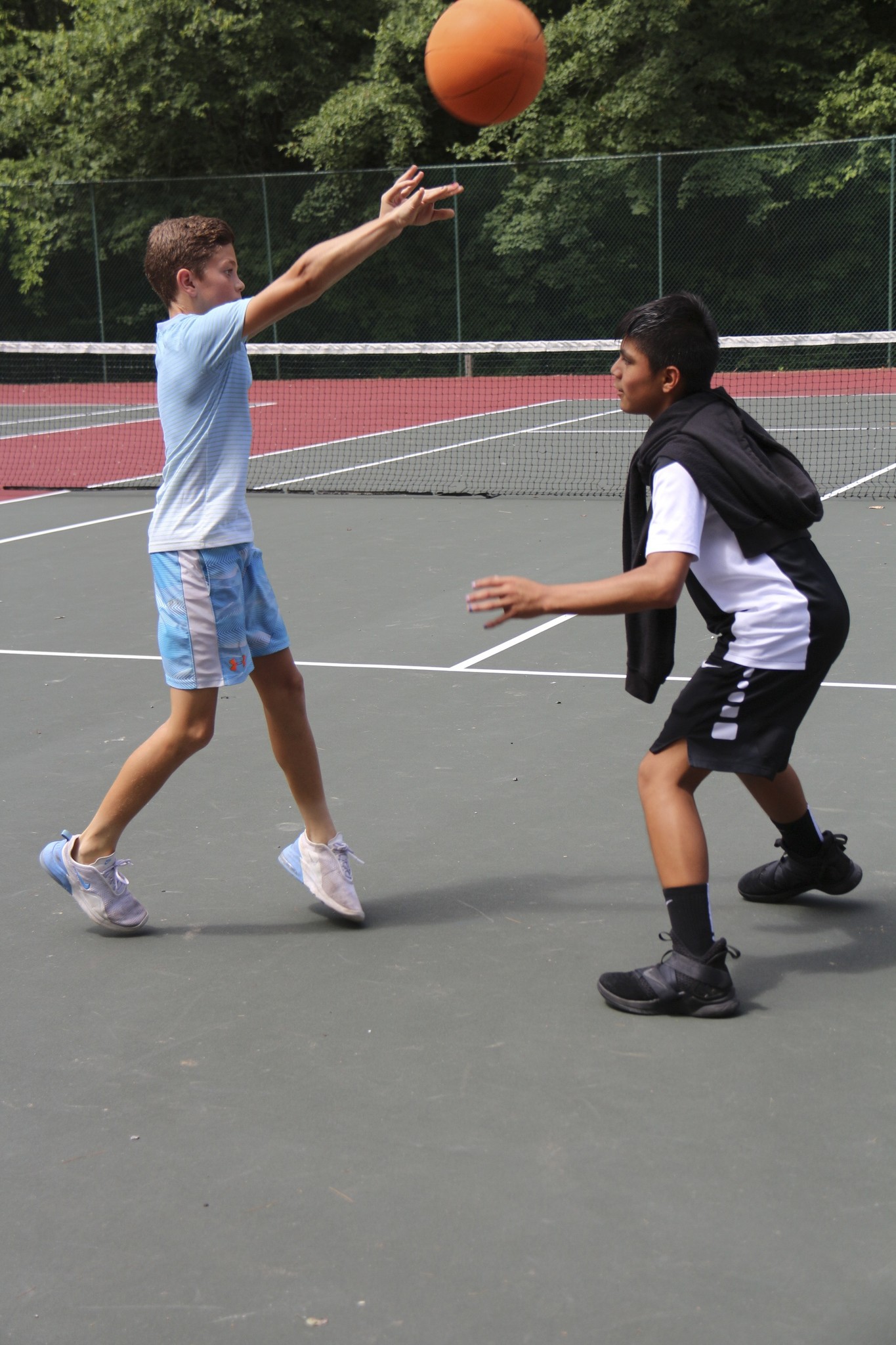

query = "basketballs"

[{"left": 422, "top": 0, "right": 549, "bottom": 126}]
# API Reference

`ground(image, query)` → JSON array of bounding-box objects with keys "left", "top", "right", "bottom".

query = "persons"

[
  {"left": 38, "top": 163, "right": 463, "bottom": 932},
  {"left": 466, "top": 289, "right": 864, "bottom": 1017}
]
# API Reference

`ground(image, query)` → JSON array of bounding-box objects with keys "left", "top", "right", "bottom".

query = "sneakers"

[
  {"left": 737, "top": 830, "right": 862, "bottom": 901},
  {"left": 277, "top": 830, "right": 366, "bottom": 925},
  {"left": 596, "top": 936, "right": 739, "bottom": 1018},
  {"left": 37, "top": 828, "right": 149, "bottom": 932}
]
